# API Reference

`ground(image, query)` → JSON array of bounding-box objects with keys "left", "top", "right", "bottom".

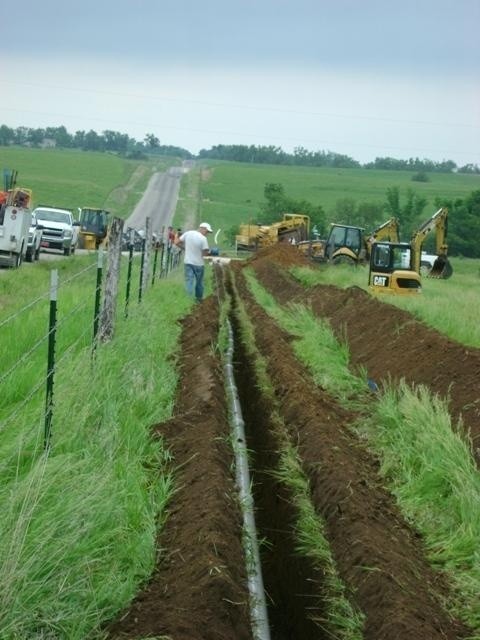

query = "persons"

[
  {"left": 167, "top": 227, "right": 174, "bottom": 253},
  {"left": 174, "top": 222, "right": 214, "bottom": 302},
  {"left": 367, "top": 235, "right": 378, "bottom": 248},
  {"left": 288, "top": 236, "right": 296, "bottom": 245},
  {"left": 175, "top": 228, "right": 182, "bottom": 238}
]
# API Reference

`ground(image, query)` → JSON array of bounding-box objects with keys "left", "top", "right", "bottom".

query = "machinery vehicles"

[
  {"left": 0, "top": 168, "right": 33, "bottom": 269},
  {"left": 235, "top": 207, "right": 453, "bottom": 295},
  {"left": 78, "top": 206, "right": 148, "bottom": 252}
]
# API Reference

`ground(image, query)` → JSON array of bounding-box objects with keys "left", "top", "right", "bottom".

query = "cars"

[{"left": 25, "top": 204, "right": 80, "bottom": 262}]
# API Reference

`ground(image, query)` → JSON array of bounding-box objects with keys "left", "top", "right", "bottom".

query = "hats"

[{"left": 200, "top": 223, "right": 213, "bottom": 233}]
